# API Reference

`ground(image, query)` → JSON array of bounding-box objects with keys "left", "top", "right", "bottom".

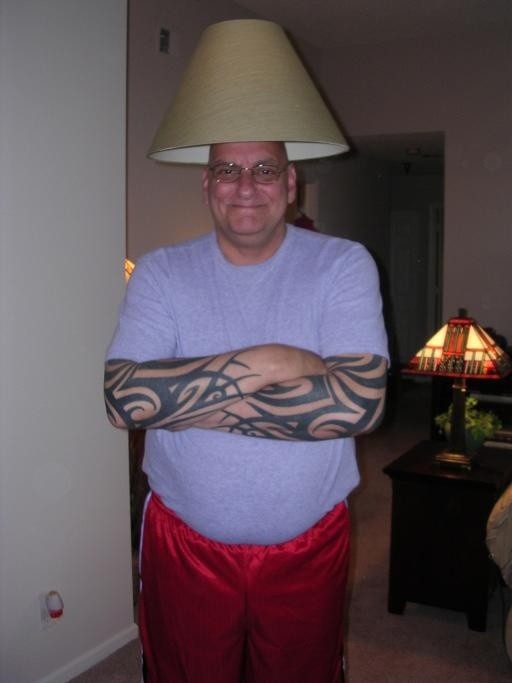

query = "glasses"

[{"left": 205, "top": 161, "right": 293, "bottom": 189}]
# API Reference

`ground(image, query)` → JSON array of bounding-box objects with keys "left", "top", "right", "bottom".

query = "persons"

[{"left": 103, "top": 138, "right": 394, "bottom": 681}]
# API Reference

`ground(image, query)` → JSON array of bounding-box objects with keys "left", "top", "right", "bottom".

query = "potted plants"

[{"left": 434, "top": 397, "right": 504, "bottom": 468}]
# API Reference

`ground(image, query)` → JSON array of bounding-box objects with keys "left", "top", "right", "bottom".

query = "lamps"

[{"left": 400, "top": 308, "right": 511, "bottom": 477}]
